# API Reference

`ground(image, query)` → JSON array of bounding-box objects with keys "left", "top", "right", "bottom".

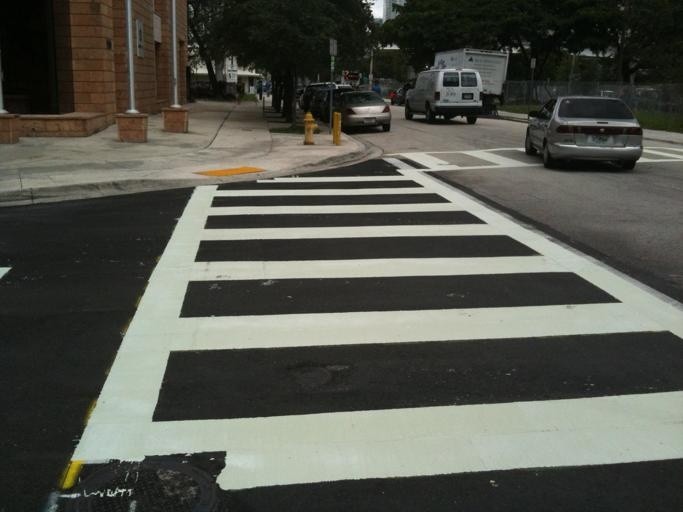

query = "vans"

[{"left": 404, "top": 66, "right": 484, "bottom": 124}]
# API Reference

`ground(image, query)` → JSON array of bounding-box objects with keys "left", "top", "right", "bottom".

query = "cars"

[
  {"left": 523, "top": 94, "right": 644, "bottom": 173},
  {"left": 296, "top": 81, "right": 391, "bottom": 134},
  {"left": 392, "top": 87, "right": 404, "bottom": 105}
]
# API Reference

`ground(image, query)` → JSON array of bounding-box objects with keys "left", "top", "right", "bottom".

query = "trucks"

[{"left": 427, "top": 48, "right": 510, "bottom": 118}]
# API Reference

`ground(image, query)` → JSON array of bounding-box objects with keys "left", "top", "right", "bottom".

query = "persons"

[
  {"left": 370, "top": 78, "right": 379, "bottom": 94},
  {"left": 258, "top": 81, "right": 262, "bottom": 100},
  {"left": 249, "top": 84, "right": 256, "bottom": 93}
]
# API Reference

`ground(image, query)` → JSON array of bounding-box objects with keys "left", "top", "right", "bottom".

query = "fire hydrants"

[{"left": 303, "top": 111, "right": 319, "bottom": 146}]
